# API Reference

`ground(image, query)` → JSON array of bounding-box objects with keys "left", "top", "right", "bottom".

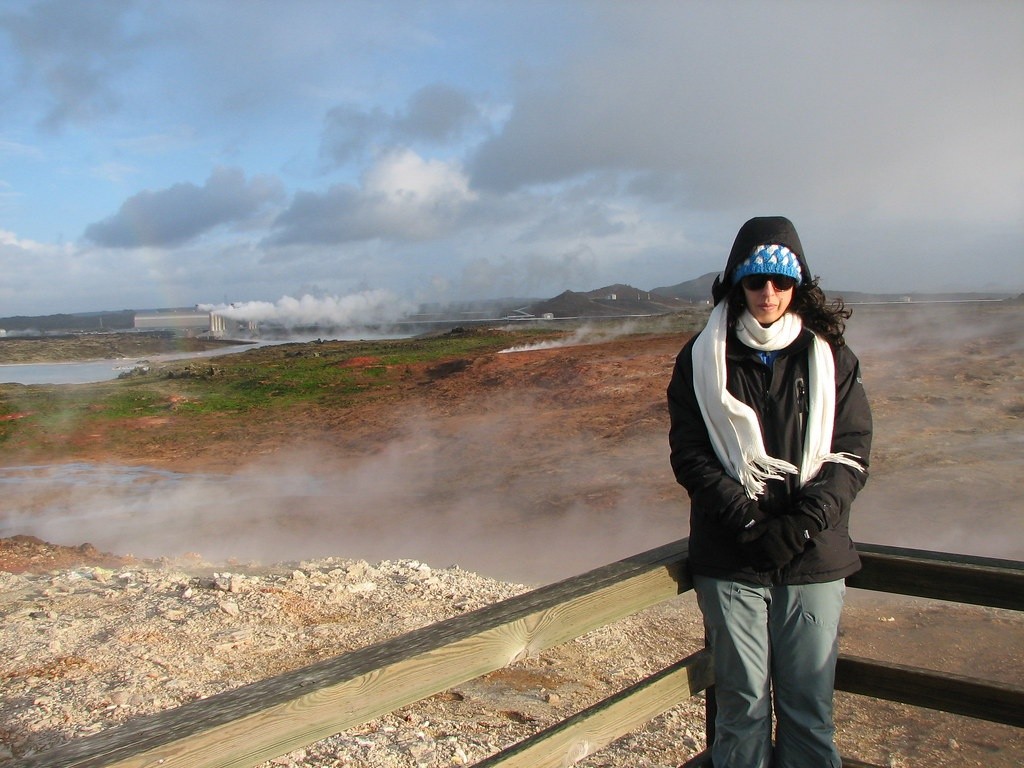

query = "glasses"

[{"left": 744, "top": 273, "right": 795, "bottom": 292}]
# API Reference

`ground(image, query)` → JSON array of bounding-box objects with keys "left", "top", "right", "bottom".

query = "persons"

[{"left": 666, "top": 216, "right": 872, "bottom": 768}]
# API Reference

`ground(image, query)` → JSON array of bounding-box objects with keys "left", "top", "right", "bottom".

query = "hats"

[{"left": 731, "top": 243, "right": 802, "bottom": 286}]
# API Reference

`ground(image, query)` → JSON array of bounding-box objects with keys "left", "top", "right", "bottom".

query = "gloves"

[{"left": 736, "top": 508, "right": 821, "bottom": 573}]
